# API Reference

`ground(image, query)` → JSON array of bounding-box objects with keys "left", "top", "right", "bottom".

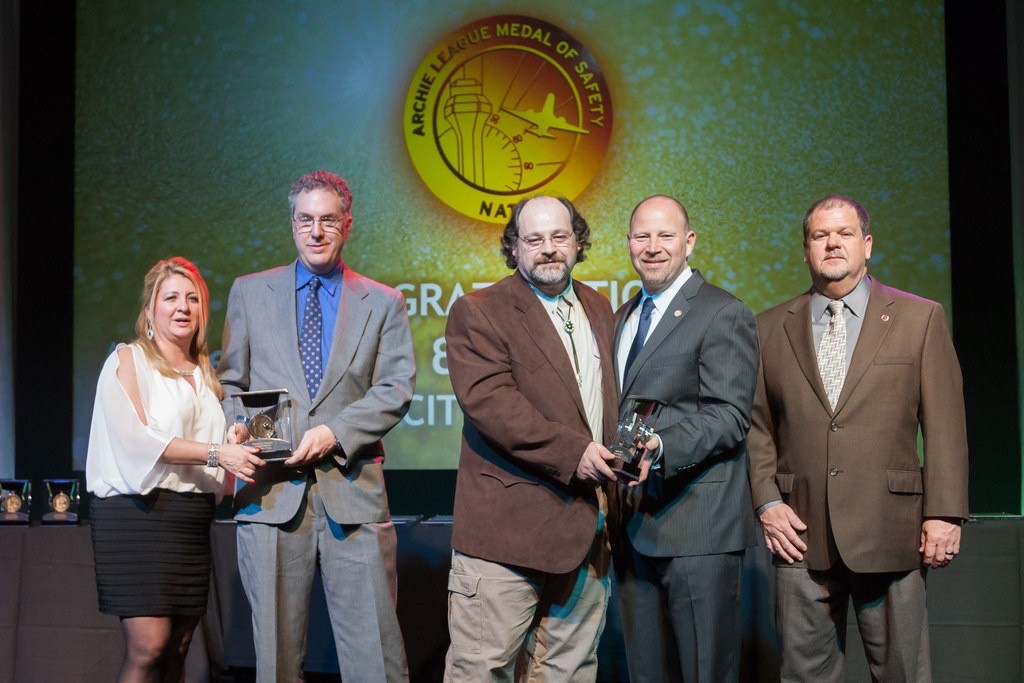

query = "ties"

[
  {"left": 623, "top": 297, "right": 655, "bottom": 395},
  {"left": 297, "top": 276, "right": 325, "bottom": 402},
  {"left": 817, "top": 298, "right": 847, "bottom": 411}
]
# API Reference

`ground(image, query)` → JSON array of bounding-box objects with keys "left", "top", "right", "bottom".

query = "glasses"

[
  {"left": 292, "top": 215, "right": 345, "bottom": 227},
  {"left": 515, "top": 231, "right": 576, "bottom": 245}
]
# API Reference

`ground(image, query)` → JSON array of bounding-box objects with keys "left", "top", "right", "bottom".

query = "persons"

[
  {"left": 744, "top": 196, "right": 969, "bottom": 683},
  {"left": 85, "top": 257, "right": 266, "bottom": 683},
  {"left": 611, "top": 194, "right": 760, "bottom": 683},
  {"left": 215, "top": 168, "right": 415, "bottom": 683},
  {"left": 440, "top": 198, "right": 621, "bottom": 683}
]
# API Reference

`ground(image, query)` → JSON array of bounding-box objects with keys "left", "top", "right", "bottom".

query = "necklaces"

[{"left": 167, "top": 363, "right": 196, "bottom": 377}]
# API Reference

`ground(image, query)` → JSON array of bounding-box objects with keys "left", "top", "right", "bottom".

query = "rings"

[{"left": 946, "top": 551, "right": 954, "bottom": 555}]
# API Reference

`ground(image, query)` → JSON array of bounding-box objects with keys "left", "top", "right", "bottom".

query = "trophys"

[
  {"left": 42, "top": 479, "right": 80, "bottom": 523},
  {"left": 230, "top": 389, "right": 294, "bottom": 462},
  {"left": 0, "top": 478, "right": 33, "bottom": 523},
  {"left": 605, "top": 394, "right": 668, "bottom": 481}
]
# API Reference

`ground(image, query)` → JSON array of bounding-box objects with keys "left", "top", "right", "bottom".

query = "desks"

[{"left": 0, "top": 514, "right": 1024, "bottom": 683}]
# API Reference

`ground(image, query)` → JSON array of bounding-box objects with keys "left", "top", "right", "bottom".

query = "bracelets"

[{"left": 206, "top": 443, "right": 220, "bottom": 468}]
